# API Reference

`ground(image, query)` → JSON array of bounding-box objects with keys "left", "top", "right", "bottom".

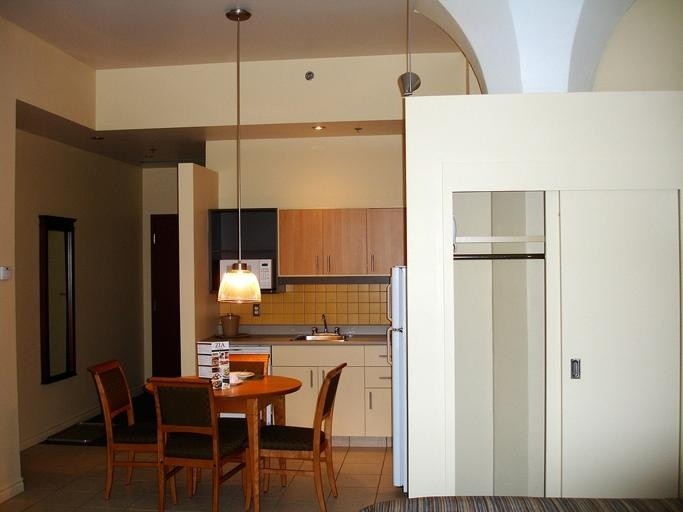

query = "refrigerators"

[{"left": 385, "top": 265, "right": 408, "bottom": 494}]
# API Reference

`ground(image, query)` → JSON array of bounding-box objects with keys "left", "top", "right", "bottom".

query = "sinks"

[{"left": 291, "top": 331, "right": 348, "bottom": 343}]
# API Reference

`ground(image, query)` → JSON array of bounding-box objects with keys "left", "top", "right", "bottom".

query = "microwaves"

[{"left": 220, "top": 259, "right": 272, "bottom": 290}]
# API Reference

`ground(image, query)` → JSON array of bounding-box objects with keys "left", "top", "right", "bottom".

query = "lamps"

[{"left": 216, "top": 10, "right": 261, "bottom": 306}]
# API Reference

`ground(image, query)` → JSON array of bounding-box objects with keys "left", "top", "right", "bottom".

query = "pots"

[{"left": 220, "top": 312, "right": 240, "bottom": 337}]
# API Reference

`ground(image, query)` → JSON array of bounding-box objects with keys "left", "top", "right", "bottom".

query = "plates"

[
  {"left": 212, "top": 332, "right": 249, "bottom": 341},
  {"left": 229, "top": 379, "right": 243, "bottom": 385},
  {"left": 229, "top": 371, "right": 256, "bottom": 380}
]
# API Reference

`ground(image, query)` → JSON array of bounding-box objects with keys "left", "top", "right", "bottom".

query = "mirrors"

[{"left": 37, "top": 213, "right": 78, "bottom": 386}]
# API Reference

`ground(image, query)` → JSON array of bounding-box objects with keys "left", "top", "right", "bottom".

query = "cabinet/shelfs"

[
  {"left": 366, "top": 208, "right": 404, "bottom": 275},
  {"left": 272, "top": 366, "right": 365, "bottom": 437},
  {"left": 278, "top": 208, "right": 366, "bottom": 275},
  {"left": 363, "top": 346, "right": 391, "bottom": 436}
]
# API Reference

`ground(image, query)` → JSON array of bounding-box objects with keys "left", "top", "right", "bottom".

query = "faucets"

[{"left": 320, "top": 313, "right": 328, "bottom": 332}]
